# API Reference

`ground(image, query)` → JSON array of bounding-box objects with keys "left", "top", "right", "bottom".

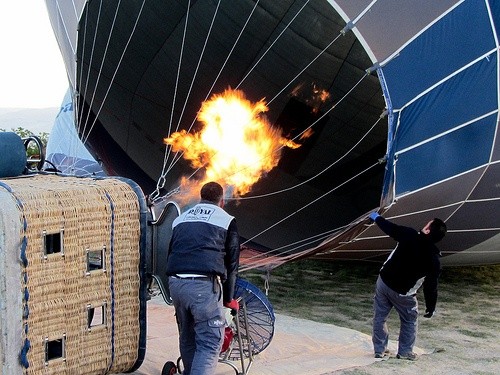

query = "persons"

[
  {"left": 163, "top": 182, "right": 240, "bottom": 375},
  {"left": 369, "top": 211, "right": 448, "bottom": 360}
]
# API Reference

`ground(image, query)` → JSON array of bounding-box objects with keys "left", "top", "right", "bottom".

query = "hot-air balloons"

[{"left": 0, "top": 0, "right": 499, "bottom": 374}]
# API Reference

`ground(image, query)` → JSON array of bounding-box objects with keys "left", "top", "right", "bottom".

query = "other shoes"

[
  {"left": 396, "top": 352, "right": 418, "bottom": 361},
  {"left": 375, "top": 349, "right": 391, "bottom": 358}
]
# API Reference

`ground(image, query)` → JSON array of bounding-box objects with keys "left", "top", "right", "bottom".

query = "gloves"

[
  {"left": 225, "top": 299, "right": 239, "bottom": 316},
  {"left": 423, "top": 308, "right": 435, "bottom": 318},
  {"left": 369, "top": 212, "right": 380, "bottom": 220}
]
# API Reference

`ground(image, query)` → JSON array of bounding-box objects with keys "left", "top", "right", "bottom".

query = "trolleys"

[{"left": 161, "top": 277, "right": 274, "bottom": 375}]
